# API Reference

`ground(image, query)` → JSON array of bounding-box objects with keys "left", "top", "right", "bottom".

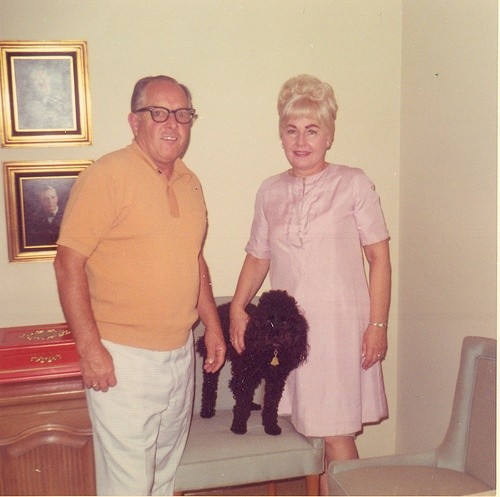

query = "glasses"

[{"left": 136, "top": 105, "right": 196, "bottom": 125}]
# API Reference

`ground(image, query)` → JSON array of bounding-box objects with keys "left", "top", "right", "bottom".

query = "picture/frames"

[
  {"left": 3, "top": 159, "right": 95, "bottom": 262},
  {"left": 0, "top": 40, "right": 95, "bottom": 147}
]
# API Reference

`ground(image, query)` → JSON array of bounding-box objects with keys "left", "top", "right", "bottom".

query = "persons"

[
  {"left": 52, "top": 76, "right": 227, "bottom": 496},
  {"left": 28, "top": 186, "right": 65, "bottom": 246},
  {"left": 230, "top": 74, "right": 391, "bottom": 496}
]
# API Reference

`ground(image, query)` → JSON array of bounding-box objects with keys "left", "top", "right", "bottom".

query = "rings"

[
  {"left": 378, "top": 355, "right": 380, "bottom": 356},
  {"left": 92, "top": 384, "right": 99, "bottom": 387}
]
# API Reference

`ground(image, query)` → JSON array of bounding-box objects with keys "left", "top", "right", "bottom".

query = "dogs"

[{"left": 196, "top": 290, "right": 309, "bottom": 436}]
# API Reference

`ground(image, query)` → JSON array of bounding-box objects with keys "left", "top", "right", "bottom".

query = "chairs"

[
  {"left": 172, "top": 296, "right": 325, "bottom": 496},
  {"left": 327, "top": 335, "right": 496, "bottom": 496}
]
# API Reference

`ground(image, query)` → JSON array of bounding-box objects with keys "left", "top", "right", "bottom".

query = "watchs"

[{"left": 369, "top": 320, "right": 388, "bottom": 328}]
